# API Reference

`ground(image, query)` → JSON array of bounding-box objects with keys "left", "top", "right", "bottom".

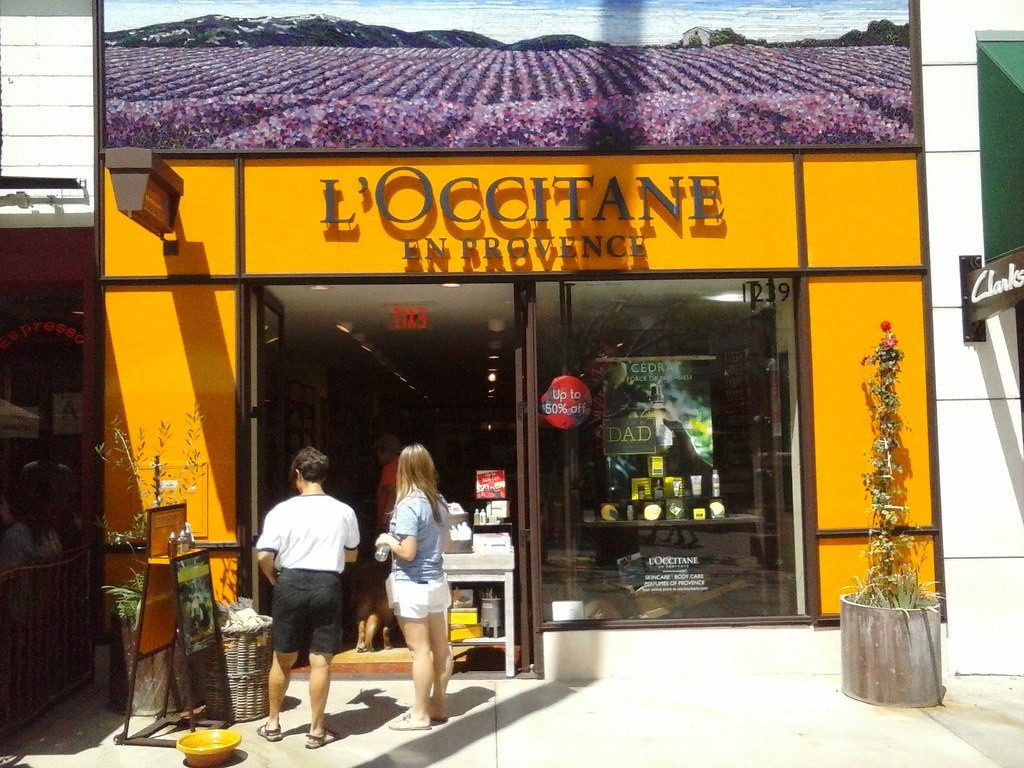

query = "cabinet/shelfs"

[{"left": 441, "top": 554, "right": 518, "bottom": 680}]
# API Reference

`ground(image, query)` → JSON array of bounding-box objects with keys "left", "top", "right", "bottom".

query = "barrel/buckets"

[{"left": 480, "top": 598, "right": 502, "bottom": 627}]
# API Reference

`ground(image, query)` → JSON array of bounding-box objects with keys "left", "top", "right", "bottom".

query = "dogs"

[{"left": 354, "top": 585, "right": 394, "bottom": 653}]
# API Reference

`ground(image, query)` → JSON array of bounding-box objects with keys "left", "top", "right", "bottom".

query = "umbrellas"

[{"left": 0, "top": 397, "right": 42, "bottom": 440}]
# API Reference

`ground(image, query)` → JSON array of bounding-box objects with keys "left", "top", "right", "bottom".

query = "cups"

[{"left": 638, "top": 486, "right": 645, "bottom": 500}]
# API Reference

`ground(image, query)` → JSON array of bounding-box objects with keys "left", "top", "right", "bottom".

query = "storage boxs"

[
  {"left": 448, "top": 626, "right": 484, "bottom": 642},
  {"left": 448, "top": 608, "right": 479, "bottom": 624}
]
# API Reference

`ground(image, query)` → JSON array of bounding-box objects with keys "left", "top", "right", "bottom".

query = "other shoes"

[{"left": 357, "top": 644, "right": 374, "bottom": 653}]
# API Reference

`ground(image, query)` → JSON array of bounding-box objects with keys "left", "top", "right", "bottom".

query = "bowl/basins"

[{"left": 176, "top": 729, "right": 242, "bottom": 767}]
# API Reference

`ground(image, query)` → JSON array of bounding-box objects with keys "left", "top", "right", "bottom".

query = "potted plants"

[{"left": 94, "top": 402, "right": 188, "bottom": 718}]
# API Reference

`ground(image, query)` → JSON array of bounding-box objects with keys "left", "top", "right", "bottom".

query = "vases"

[{"left": 841, "top": 591, "right": 951, "bottom": 708}]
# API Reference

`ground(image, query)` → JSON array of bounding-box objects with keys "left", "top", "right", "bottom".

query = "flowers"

[{"left": 858, "top": 317, "right": 943, "bottom": 608}]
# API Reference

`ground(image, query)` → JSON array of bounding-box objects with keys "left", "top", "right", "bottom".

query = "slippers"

[
  {"left": 403, "top": 712, "right": 448, "bottom": 725},
  {"left": 389, "top": 713, "right": 432, "bottom": 731}
]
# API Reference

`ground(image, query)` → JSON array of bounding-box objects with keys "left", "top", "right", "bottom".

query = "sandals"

[
  {"left": 257, "top": 722, "right": 283, "bottom": 742},
  {"left": 305, "top": 727, "right": 341, "bottom": 749}
]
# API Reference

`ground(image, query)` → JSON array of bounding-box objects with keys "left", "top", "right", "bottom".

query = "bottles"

[
  {"left": 480, "top": 509, "right": 486, "bottom": 525},
  {"left": 711, "top": 469, "right": 721, "bottom": 496},
  {"left": 375, "top": 532, "right": 393, "bottom": 561},
  {"left": 655, "top": 480, "right": 663, "bottom": 499},
  {"left": 474, "top": 509, "right": 480, "bottom": 525}
]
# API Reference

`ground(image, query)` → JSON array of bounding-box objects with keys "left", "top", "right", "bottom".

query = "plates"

[
  {"left": 644, "top": 505, "right": 662, "bottom": 519},
  {"left": 602, "top": 505, "right": 619, "bottom": 521},
  {"left": 709, "top": 502, "right": 725, "bottom": 517}
]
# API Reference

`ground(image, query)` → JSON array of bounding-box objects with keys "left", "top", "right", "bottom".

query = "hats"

[{"left": 369, "top": 434, "right": 400, "bottom": 454}]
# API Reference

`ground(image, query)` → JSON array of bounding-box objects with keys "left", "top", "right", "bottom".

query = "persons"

[
  {"left": 348, "top": 434, "right": 403, "bottom": 651},
  {"left": 255, "top": 446, "right": 360, "bottom": 749},
  {"left": 0, "top": 487, "right": 67, "bottom": 612},
  {"left": 607, "top": 381, "right": 713, "bottom": 498},
  {"left": 374, "top": 442, "right": 453, "bottom": 731},
  {"left": 642, "top": 527, "right": 698, "bottom": 547}
]
